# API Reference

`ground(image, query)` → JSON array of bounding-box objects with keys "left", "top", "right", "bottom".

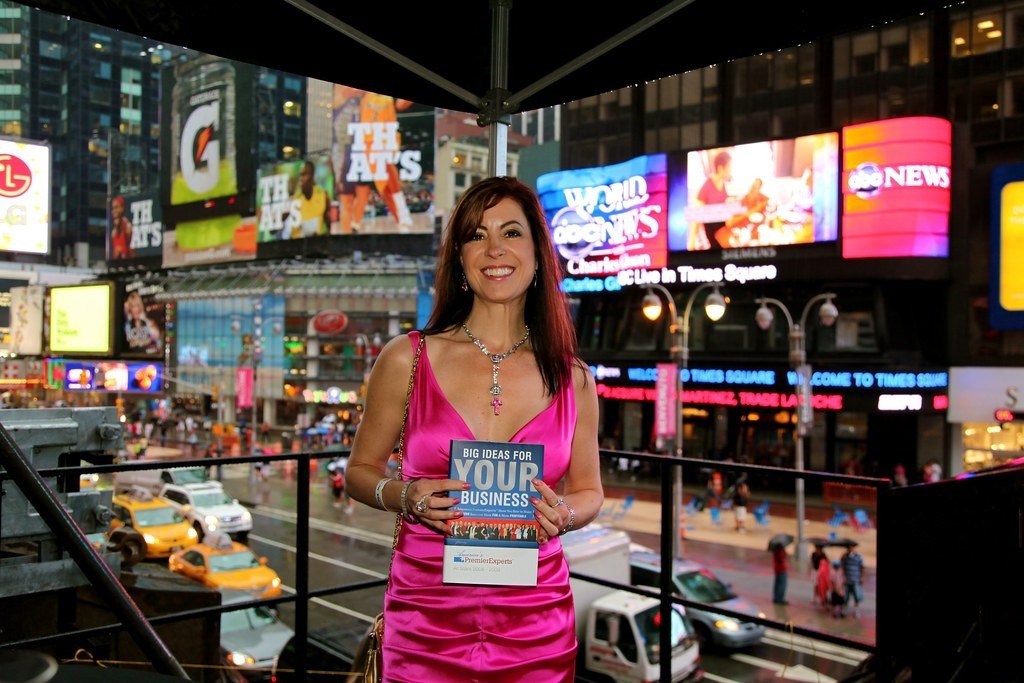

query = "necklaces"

[{"left": 460, "top": 320, "right": 529, "bottom": 414}]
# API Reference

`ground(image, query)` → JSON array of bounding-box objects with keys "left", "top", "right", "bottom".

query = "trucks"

[{"left": 552, "top": 523, "right": 701, "bottom": 682}]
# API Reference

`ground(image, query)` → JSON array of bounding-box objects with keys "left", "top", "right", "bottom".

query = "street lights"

[
  {"left": 755, "top": 293, "right": 842, "bottom": 581},
  {"left": 640, "top": 283, "right": 727, "bottom": 564}
]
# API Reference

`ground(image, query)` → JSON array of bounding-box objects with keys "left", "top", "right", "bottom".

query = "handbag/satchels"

[{"left": 365, "top": 612, "right": 383, "bottom": 683}]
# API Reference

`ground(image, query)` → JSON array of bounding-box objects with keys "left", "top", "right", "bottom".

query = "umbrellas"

[
  {"left": 833, "top": 537, "right": 860, "bottom": 548},
  {"left": 803, "top": 536, "right": 832, "bottom": 548},
  {"left": 765, "top": 532, "right": 794, "bottom": 552}
]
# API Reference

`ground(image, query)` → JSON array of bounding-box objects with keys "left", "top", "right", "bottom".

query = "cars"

[
  {"left": 212, "top": 588, "right": 295, "bottom": 682},
  {"left": 161, "top": 467, "right": 208, "bottom": 484},
  {"left": 111, "top": 483, "right": 199, "bottom": 558},
  {"left": 273, "top": 621, "right": 371, "bottom": 683},
  {"left": 631, "top": 550, "right": 767, "bottom": 655},
  {"left": 169, "top": 528, "right": 282, "bottom": 599}
]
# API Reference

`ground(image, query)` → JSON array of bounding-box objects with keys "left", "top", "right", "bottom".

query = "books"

[{"left": 442, "top": 440, "right": 545, "bottom": 588}]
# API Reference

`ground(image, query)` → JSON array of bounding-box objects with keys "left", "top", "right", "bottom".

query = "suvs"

[{"left": 158, "top": 480, "right": 252, "bottom": 541}]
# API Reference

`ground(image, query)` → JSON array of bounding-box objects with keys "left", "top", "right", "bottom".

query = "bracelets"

[
  {"left": 559, "top": 503, "right": 575, "bottom": 536},
  {"left": 375, "top": 478, "right": 395, "bottom": 512},
  {"left": 399, "top": 480, "right": 420, "bottom": 525}
]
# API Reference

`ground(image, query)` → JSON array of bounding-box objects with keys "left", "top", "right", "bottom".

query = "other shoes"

[{"left": 772, "top": 598, "right": 790, "bottom": 606}]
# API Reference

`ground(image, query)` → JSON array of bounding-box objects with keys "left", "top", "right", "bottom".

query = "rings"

[
  {"left": 415, "top": 495, "right": 428, "bottom": 512},
  {"left": 552, "top": 500, "right": 564, "bottom": 508}
]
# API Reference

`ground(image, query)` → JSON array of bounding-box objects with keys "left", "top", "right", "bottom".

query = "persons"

[
  {"left": 329, "top": 85, "right": 416, "bottom": 231},
  {"left": 344, "top": 175, "right": 604, "bottom": 683},
  {"left": 109, "top": 198, "right": 136, "bottom": 260},
  {"left": 829, "top": 560, "right": 848, "bottom": 619},
  {"left": 687, "top": 152, "right": 770, "bottom": 253},
  {"left": 810, "top": 546, "right": 830, "bottom": 605},
  {"left": 772, "top": 549, "right": 792, "bottom": 604},
  {"left": 286, "top": 162, "right": 332, "bottom": 237},
  {"left": 734, "top": 483, "right": 750, "bottom": 535},
  {"left": 449, "top": 520, "right": 539, "bottom": 541},
  {"left": 123, "top": 399, "right": 723, "bottom": 517},
  {"left": 840, "top": 547, "right": 866, "bottom": 618},
  {"left": 121, "top": 294, "right": 158, "bottom": 354}
]
